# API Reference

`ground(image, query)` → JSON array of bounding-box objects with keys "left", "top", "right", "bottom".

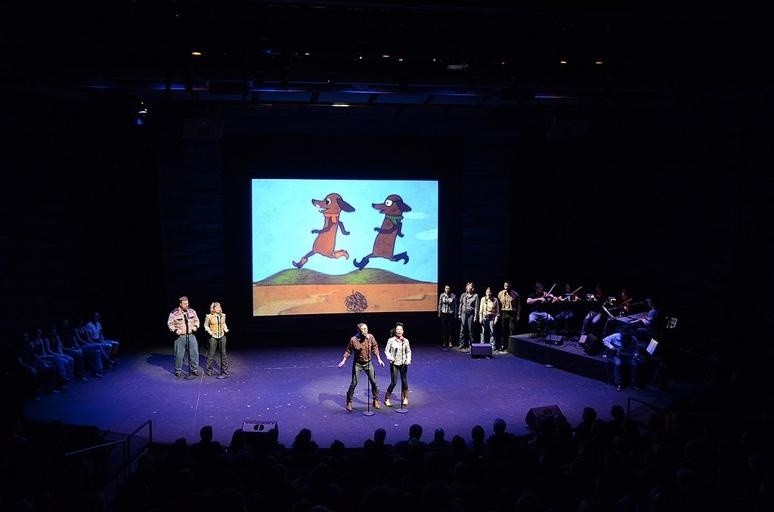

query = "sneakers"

[
  {"left": 205, "top": 370, "right": 212, "bottom": 375},
  {"left": 193, "top": 371, "right": 199, "bottom": 375},
  {"left": 223, "top": 371, "right": 230, "bottom": 375},
  {"left": 175, "top": 373, "right": 181, "bottom": 376}
]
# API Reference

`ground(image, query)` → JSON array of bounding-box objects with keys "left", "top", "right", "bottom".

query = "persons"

[
  {"left": 166, "top": 295, "right": 204, "bottom": 378},
  {"left": 202, "top": 301, "right": 230, "bottom": 377},
  {"left": 336, "top": 322, "right": 387, "bottom": 412},
  {"left": 436, "top": 280, "right": 659, "bottom": 358},
  {"left": 15, "top": 312, "right": 120, "bottom": 402},
  {"left": 131, "top": 404, "right": 773, "bottom": 512},
  {"left": 601, "top": 324, "right": 642, "bottom": 393},
  {"left": 383, "top": 320, "right": 412, "bottom": 408}
]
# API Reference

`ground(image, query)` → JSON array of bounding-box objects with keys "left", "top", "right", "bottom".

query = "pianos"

[{"left": 615, "top": 312, "right": 649, "bottom": 330}]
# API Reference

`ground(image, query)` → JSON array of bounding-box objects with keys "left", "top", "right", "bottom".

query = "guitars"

[{"left": 605, "top": 341, "right": 645, "bottom": 363}]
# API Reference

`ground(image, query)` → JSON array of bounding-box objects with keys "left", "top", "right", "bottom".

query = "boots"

[
  {"left": 353, "top": 257, "right": 369, "bottom": 270},
  {"left": 335, "top": 250, "right": 349, "bottom": 259},
  {"left": 395, "top": 251, "right": 409, "bottom": 264},
  {"left": 292, "top": 256, "right": 308, "bottom": 268}
]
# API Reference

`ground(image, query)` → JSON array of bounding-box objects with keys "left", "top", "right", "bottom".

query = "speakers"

[
  {"left": 525, "top": 406, "right": 567, "bottom": 428},
  {"left": 471, "top": 347, "right": 492, "bottom": 358},
  {"left": 241, "top": 420, "right": 279, "bottom": 441}
]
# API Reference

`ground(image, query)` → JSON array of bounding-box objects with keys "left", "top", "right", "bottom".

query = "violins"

[{"left": 537, "top": 292, "right": 632, "bottom": 314}]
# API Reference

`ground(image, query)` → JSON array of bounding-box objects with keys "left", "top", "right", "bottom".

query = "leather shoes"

[
  {"left": 403, "top": 398, "right": 408, "bottom": 405},
  {"left": 385, "top": 399, "right": 391, "bottom": 406},
  {"left": 617, "top": 384, "right": 622, "bottom": 391},
  {"left": 346, "top": 401, "right": 353, "bottom": 411},
  {"left": 443, "top": 343, "right": 506, "bottom": 351},
  {"left": 373, "top": 400, "right": 380, "bottom": 409},
  {"left": 633, "top": 386, "right": 639, "bottom": 390}
]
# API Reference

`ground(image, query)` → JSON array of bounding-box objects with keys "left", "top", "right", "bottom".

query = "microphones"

[{"left": 184, "top": 314, "right": 187, "bottom": 322}]
